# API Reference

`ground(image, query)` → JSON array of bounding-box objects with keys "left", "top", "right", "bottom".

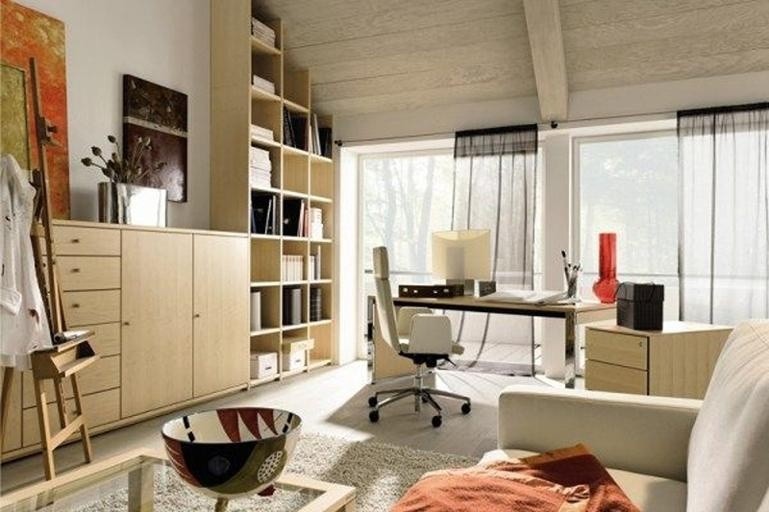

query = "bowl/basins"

[{"left": 160, "top": 407, "right": 302, "bottom": 500}]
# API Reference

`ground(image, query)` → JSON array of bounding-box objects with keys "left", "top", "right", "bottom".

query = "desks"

[{"left": 366, "top": 281, "right": 616, "bottom": 393}]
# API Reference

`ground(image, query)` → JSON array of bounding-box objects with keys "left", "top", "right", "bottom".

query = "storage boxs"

[
  {"left": 282, "top": 349, "right": 306, "bottom": 372},
  {"left": 251, "top": 353, "right": 278, "bottom": 379},
  {"left": 310, "top": 223, "right": 324, "bottom": 238}
]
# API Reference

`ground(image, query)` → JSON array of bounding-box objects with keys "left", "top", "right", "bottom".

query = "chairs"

[{"left": 369, "top": 247, "right": 471, "bottom": 428}]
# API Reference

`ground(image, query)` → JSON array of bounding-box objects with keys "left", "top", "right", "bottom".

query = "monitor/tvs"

[{"left": 431, "top": 229, "right": 491, "bottom": 296}]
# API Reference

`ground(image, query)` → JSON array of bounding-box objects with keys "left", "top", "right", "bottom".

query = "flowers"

[{"left": 80, "top": 134, "right": 167, "bottom": 182}]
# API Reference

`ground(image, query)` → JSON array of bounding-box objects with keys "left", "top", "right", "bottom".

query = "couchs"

[{"left": 385, "top": 314, "right": 769, "bottom": 512}]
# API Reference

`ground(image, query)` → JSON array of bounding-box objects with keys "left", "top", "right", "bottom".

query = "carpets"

[{"left": 68, "top": 434, "right": 485, "bottom": 512}]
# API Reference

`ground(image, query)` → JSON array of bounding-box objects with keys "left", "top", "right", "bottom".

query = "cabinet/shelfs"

[
  {"left": 582, "top": 319, "right": 732, "bottom": 400},
  {"left": 206, "top": 0, "right": 341, "bottom": 391}
]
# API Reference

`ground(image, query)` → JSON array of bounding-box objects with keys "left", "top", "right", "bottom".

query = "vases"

[
  {"left": 592, "top": 232, "right": 620, "bottom": 304},
  {"left": 96, "top": 181, "right": 168, "bottom": 227}
]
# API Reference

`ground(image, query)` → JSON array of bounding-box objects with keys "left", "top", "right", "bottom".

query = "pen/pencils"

[{"left": 562, "top": 251, "right": 581, "bottom": 286}]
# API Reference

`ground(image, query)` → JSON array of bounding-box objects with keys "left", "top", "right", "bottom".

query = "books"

[{"left": 251, "top": 17, "right": 331, "bottom": 330}]
